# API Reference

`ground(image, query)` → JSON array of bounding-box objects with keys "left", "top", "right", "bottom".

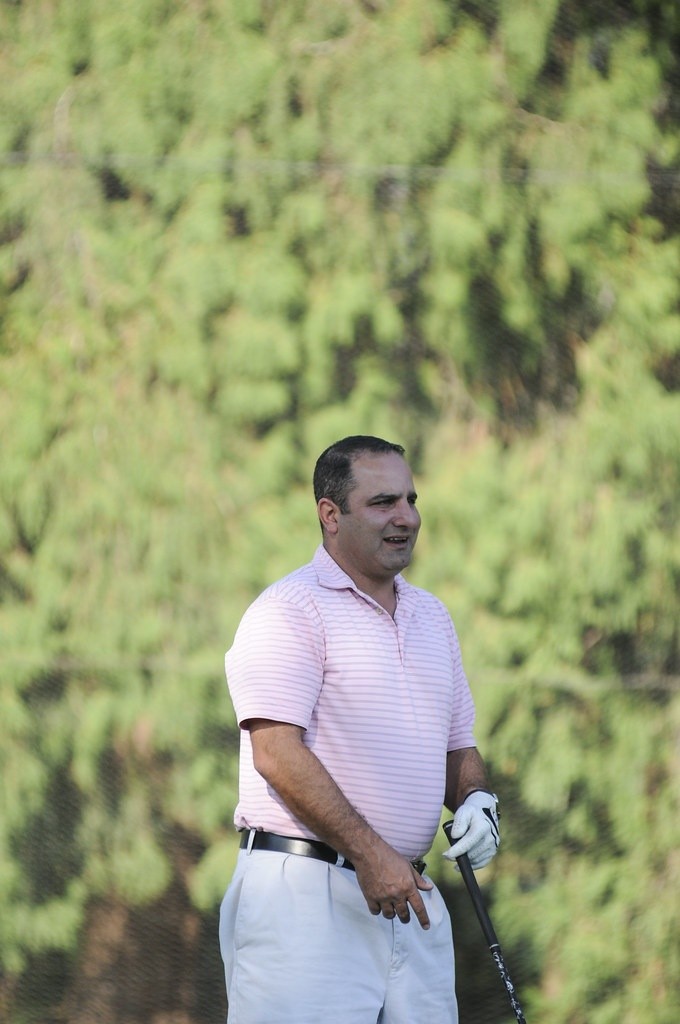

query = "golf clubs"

[{"left": 441, "top": 819, "right": 527, "bottom": 1024}]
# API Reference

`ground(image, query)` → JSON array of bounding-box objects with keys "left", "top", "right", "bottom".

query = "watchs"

[{"left": 463, "top": 789, "right": 501, "bottom": 820}]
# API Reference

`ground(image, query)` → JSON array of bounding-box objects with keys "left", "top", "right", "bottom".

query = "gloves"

[{"left": 442, "top": 789, "right": 501, "bottom": 871}]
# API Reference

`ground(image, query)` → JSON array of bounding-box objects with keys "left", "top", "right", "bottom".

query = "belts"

[{"left": 240, "top": 829, "right": 426, "bottom": 877}]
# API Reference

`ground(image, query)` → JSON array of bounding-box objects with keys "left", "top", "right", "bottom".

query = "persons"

[{"left": 219, "top": 436, "right": 501, "bottom": 1024}]
